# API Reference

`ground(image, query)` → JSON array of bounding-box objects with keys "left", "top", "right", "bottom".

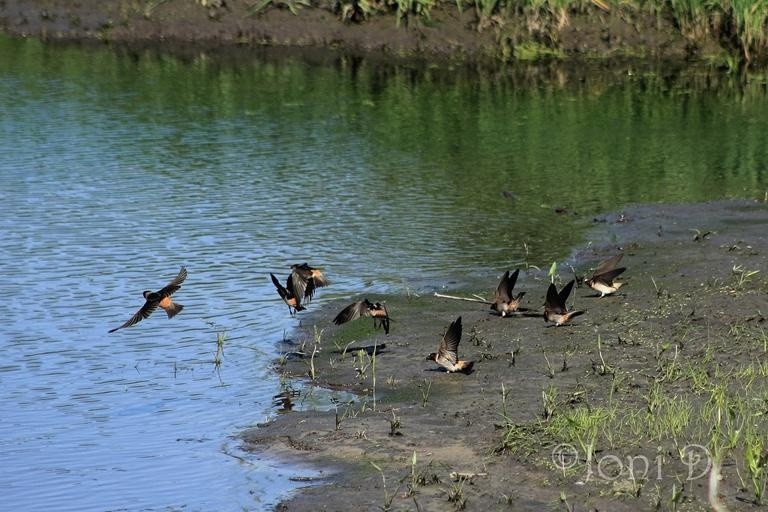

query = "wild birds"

[
  {"left": 290, "top": 263, "right": 328, "bottom": 301},
  {"left": 107, "top": 265, "right": 187, "bottom": 333},
  {"left": 426, "top": 316, "right": 475, "bottom": 375},
  {"left": 333, "top": 298, "right": 389, "bottom": 335},
  {"left": 490, "top": 269, "right": 526, "bottom": 319},
  {"left": 270, "top": 271, "right": 307, "bottom": 314},
  {"left": 584, "top": 253, "right": 628, "bottom": 299},
  {"left": 543, "top": 279, "right": 585, "bottom": 327}
]
